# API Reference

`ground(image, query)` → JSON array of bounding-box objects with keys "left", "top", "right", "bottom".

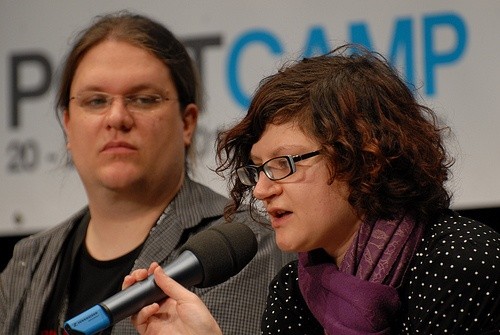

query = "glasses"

[
  {"left": 67, "top": 92, "right": 179, "bottom": 114},
  {"left": 236, "top": 147, "right": 326, "bottom": 187}
]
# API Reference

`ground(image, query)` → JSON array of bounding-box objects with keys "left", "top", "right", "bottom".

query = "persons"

[
  {"left": 121, "top": 44, "right": 500, "bottom": 335},
  {"left": 0, "top": 15, "right": 300, "bottom": 335}
]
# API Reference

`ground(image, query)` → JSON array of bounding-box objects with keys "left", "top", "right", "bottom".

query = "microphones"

[{"left": 63, "top": 224, "right": 258, "bottom": 335}]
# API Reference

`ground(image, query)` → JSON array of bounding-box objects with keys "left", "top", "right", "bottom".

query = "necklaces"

[{"left": 60, "top": 203, "right": 172, "bottom": 329}]
